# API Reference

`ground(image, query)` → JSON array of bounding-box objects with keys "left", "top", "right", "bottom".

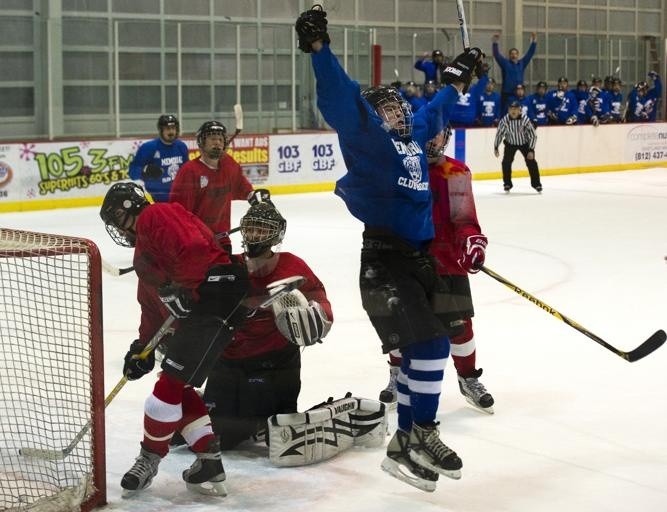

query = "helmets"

[
  {"left": 426, "top": 120, "right": 452, "bottom": 162},
  {"left": 100, "top": 182, "right": 150, "bottom": 248},
  {"left": 487, "top": 76, "right": 648, "bottom": 97},
  {"left": 506, "top": 101, "right": 522, "bottom": 120},
  {"left": 193, "top": 120, "right": 230, "bottom": 161},
  {"left": 360, "top": 83, "right": 414, "bottom": 142},
  {"left": 240, "top": 204, "right": 286, "bottom": 256},
  {"left": 405, "top": 49, "right": 442, "bottom": 92},
  {"left": 157, "top": 115, "right": 179, "bottom": 145}
]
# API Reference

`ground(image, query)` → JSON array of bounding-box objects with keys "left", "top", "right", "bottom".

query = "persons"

[
  {"left": 390, "top": 31, "right": 662, "bottom": 128},
  {"left": 493, "top": 102, "right": 543, "bottom": 193},
  {"left": 198, "top": 202, "right": 385, "bottom": 467},
  {"left": 378, "top": 123, "right": 493, "bottom": 408},
  {"left": 168, "top": 120, "right": 269, "bottom": 259},
  {"left": 294, "top": 4, "right": 480, "bottom": 481},
  {"left": 128, "top": 114, "right": 190, "bottom": 202},
  {"left": 99, "top": 182, "right": 247, "bottom": 491}
]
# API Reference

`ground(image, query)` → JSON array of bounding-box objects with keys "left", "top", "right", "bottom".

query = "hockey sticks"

[
  {"left": 477, "top": 264, "right": 667, "bottom": 362},
  {"left": 18, "top": 314, "right": 179, "bottom": 461},
  {"left": 226, "top": 103, "right": 244, "bottom": 146},
  {"left": 100, "top": 227, "right": 242, "bottom": 275}
]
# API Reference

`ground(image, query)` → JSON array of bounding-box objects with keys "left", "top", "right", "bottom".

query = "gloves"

[
  {"left": 649, "top": 69, "right": 661, "bottom": 81},
  {"left": 122, "top": 339, "right": 157, "bottom": 383},
  {"left": 248, "top": 188, "right": 275, "bottom": 206},
  {"left": 444, "top": 46, "right": 480, "bottom": 85},
  {"left": 532, "top": 112, "right": 652, "bottom": 126},
  {"left": 143, "top": 165, "right": 165, "bottom": 178},
  {"left": 455, "top": 233, "right": 489, "bottom": 275},
  {"left": 293, "top": 3, "right": 332, "bottom": 53},
  {"left": 154, "top": 286, "right": 192, "bottom": 319}
]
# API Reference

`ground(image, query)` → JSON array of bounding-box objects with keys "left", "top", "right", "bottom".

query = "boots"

[
  {"left": 456, "top": 363, "right": 495, "bottom": 405},
  {"left": 382, "top": 362, "right": 403, "bottom": 403},
  {"left": 387, "top": 427, "right": 440, "bottom": 481},
  {"left": 123, "top": 447, "right": 159, "bottom": 491},
  {"left": 178, "top": 437, "right": 229, "bottom": 484},
  {"left": 412, "top": 421, "right": 462, "bottom": 468}
]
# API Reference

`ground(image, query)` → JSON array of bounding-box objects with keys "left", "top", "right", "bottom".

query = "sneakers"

[{"left": 502, "top": 180, "right": 542, "bottom": 191}]
